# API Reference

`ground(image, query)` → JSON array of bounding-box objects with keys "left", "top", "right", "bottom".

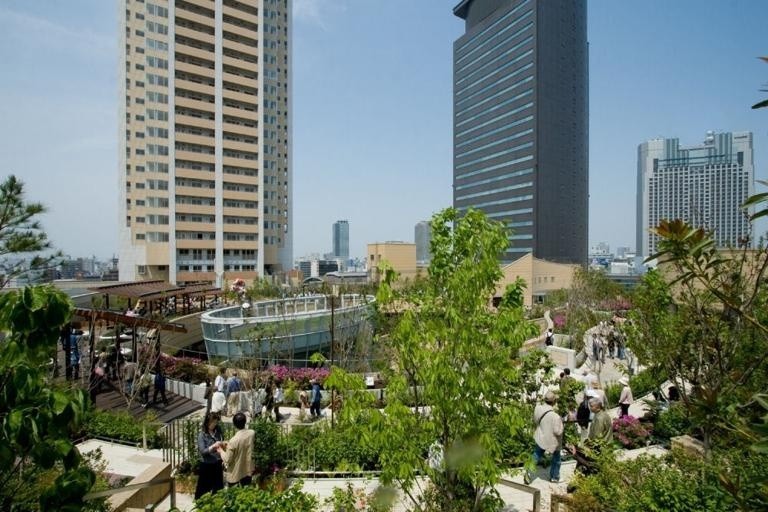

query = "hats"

[
  {"left": 619, "top": 377, "right": 629, "bottom": 386},
  {"left": 592, "top": 381, "right": 599, "bottom": 388},
  {"left": 545, "top": 391, "right": 559, "bottom": 402},
  {"left": 309, "top": 378, "right": 315, "bottom": 383}
]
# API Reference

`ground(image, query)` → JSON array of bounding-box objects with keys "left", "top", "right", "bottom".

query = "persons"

[
  {"left": 193, "top": 410, "right": 220, "bottom": 496},
  {"left": 584, "top": 395, "right": 613, "bottom": 444},
  {"left": 524, "top": 390, "right": 563, "bottom": 486},
  {"left": 216, "top": 411, "right": 255, "bottom": 490},
  {"left": 31, "top": 299, "right": 680, "bottom": 431}
]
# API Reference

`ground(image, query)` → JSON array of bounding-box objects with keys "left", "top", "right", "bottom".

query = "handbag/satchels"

[{"left": 578, "top": 402, "right": 590, "bottom": 428}]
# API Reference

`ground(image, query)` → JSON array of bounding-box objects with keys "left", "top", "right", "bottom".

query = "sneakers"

[{"left": 551, "top": 477, "right": 563, "bottom": 483}]
